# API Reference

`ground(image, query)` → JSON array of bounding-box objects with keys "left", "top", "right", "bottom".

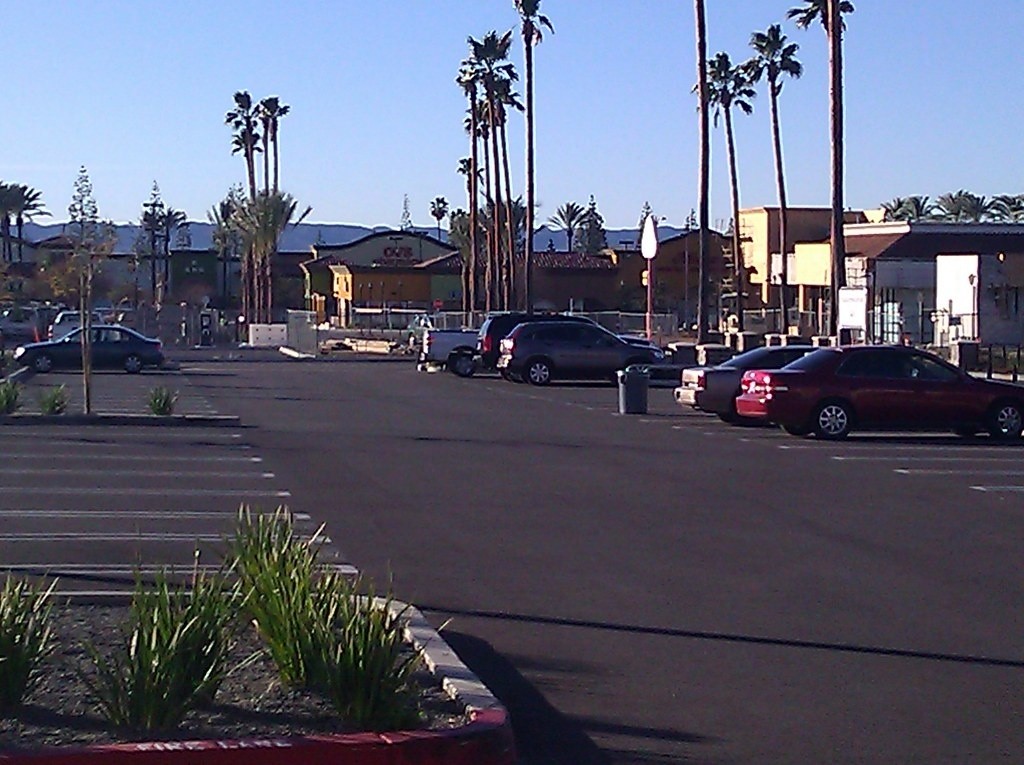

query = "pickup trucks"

[
  {"left": 422, "top": 329, "right": 480, "bottom": 377},
  {"left": 48, "top": 310, "right": 105, "bottom": 344}
]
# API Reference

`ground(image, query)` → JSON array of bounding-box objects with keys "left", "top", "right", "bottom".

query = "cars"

[
  {"left": 13, "top": 324, "right": 165, "bottom": 374},
  {"left": 736, "top": 345, "right": 1024, "bottom": 443},
  {"left": 674, "top": 346, "right": 819, "bottom": 429}
]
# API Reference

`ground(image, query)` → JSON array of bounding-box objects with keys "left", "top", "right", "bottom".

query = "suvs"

[
  {"left": 96, "top": 306, "right": 137, "bottom": 331},
  {"left": 1, "top": 305, "right": 58, "bottom": 344},
  {"left": 496, "top": 321, "right": 667, "bottom": 387},
  {"left": 473, "top": 312, "right": 657, "bottom": 384}
]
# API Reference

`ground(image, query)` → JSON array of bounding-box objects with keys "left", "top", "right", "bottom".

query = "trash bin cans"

[{"left": 615, "top": 370, "right": 652, "bottom": 414}]
